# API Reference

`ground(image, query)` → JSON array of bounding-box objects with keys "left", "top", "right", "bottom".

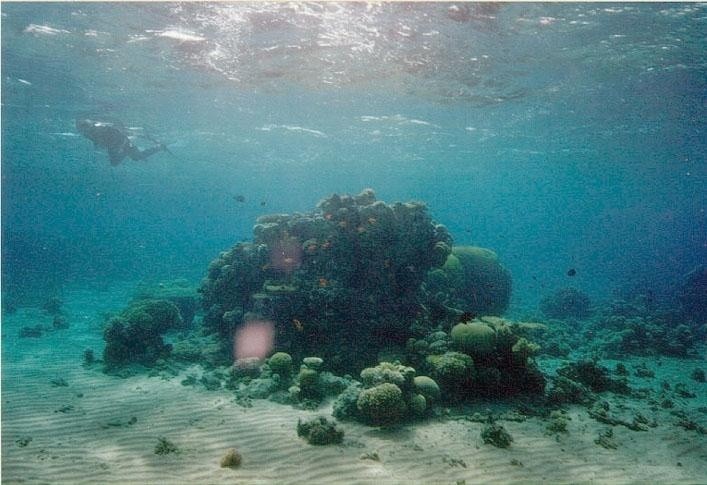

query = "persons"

[{"left": 76, "top": 118, "right": 164, "bottom": 167}]
